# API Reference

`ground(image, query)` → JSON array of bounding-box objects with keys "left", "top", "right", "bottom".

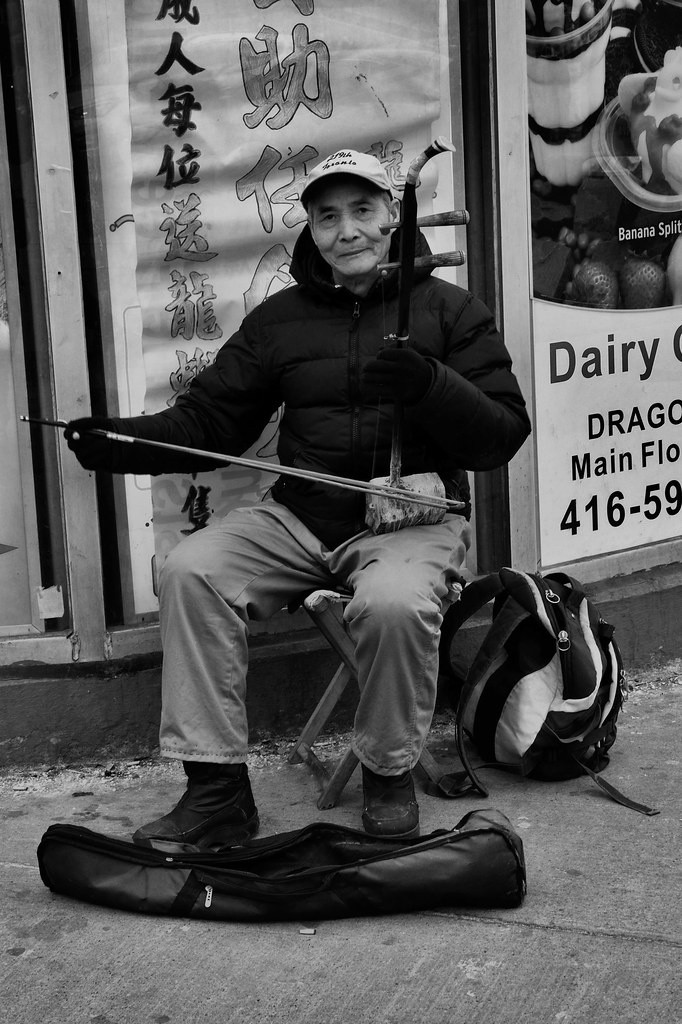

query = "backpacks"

[{"left": 440, "top": 567, "right": 623, "bottom": 798}]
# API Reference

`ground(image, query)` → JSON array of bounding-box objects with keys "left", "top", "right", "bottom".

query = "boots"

[
  {"left": 132, "top": 761, "right": 260, "bottom": 852},
  {"left": 360, "top": 762, "right": 420, "bottom": 839}
]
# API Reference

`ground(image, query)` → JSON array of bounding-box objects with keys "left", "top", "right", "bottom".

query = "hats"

[{"left": 300, "top": 150, "right": 393, "bottom": 213}]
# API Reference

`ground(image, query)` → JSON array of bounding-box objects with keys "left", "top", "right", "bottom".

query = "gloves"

[
  {"left": 364, "top": 346, "right": 430, "bottom": 402},
  {"left": 64, "top": 417, "right": 120, "bottom": 473}
]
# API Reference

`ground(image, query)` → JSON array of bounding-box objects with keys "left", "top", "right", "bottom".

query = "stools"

[{"left": 287, "top": 578, "right": 462, "bottom": 810}]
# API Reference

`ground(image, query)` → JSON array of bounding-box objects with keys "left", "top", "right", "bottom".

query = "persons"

[{"left": 62, "top": 149, "right": 530, "bottom": 848}]
